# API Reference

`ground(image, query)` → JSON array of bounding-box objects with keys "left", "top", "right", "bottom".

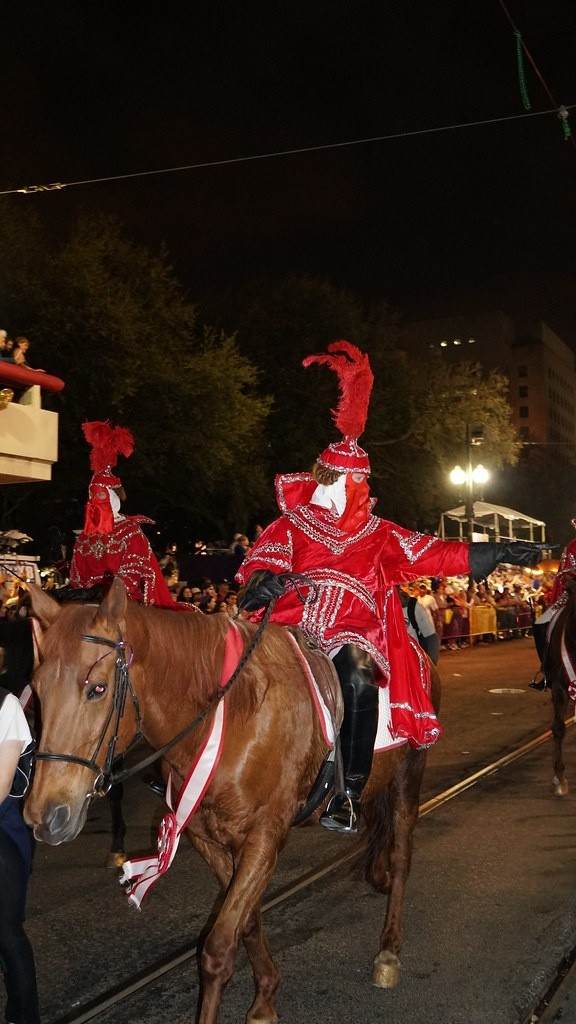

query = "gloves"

[
  {"left": 236, "top": 569, "right": 286, "bottom": 612},
  {"left": 469, "top": 540, "right": 561, "bottom": 584},
  {"left": 64, "top": 582, "right": 105, "bottom": 601}
]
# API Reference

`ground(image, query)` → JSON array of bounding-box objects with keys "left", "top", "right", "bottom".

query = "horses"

[
  {"left": 533, "top": 583, "right": 576, "bottom": 796},
  {"left": 0, "top": 569, "right": 443, "bottom": 1024}
]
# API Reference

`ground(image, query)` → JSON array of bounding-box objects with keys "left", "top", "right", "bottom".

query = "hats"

[
  {"left": 217, "top": 578, "right": 230, "bottom": 584},
  {"left": 82, "top": 421, "right": 134, "bottom": 489},
  {"left": 304, "top": 340, "right": 374, "bottom": 476}
]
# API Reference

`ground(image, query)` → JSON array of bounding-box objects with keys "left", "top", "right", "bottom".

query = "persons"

[
  {"left": 0, "top": 558, "right": 70, "bottom": 620},
  {"left": 396, "top": 528, "right": 557, "bottom": 668},
  {"left": 159, "top": 523, "right": 263, "bottom": 620},
  {"left": 0, "top": 627, "right": 37, "bottom": 1024},
  {"left": 50, "top": 469, "right": 196, "bottom": 611},
  {"left": 146, "top": 444, "right": 562, "bottom": 834},
  {"left": 527, "top": 516, "right": 576, "bottom": 692},
  {"left": 0, "top": 329, "right": 46, "bottom": 373}
]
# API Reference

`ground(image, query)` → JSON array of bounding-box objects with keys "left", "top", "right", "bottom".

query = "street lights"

[{"left": 447, "top": 464, "right": 490, "bottom": 544}]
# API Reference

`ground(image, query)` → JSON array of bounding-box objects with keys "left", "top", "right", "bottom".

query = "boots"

[
  {"left": 321, "top": 643, "right": 379, "bottom": 832},
  {"left": 146, "top": 778, "right": 167, "bottom": 798},
  {"left": 528, "top": 621, "right": 552, "bottom": 690}
]
